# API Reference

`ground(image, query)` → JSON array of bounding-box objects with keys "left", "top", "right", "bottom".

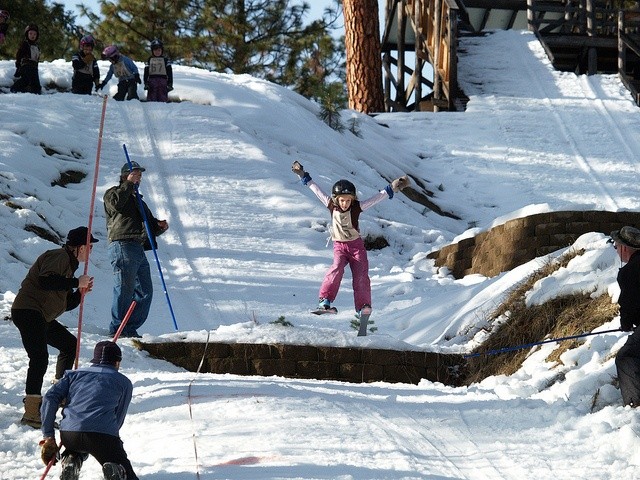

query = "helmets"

[
  {"left": 101, "top": 45, "right": 119, "bottom": 61},
  {"left": 24, "top": 24, "right": 39, "bottom": 37},
  {"left": 0, "top": 10, "right": 10, "bottom": 19},
  {"left": 151, "top": 39, "right": 164, "bottom": 49},
  {"left": 332, "top": 180, "right": 356, "bottom": 198},
  {"left": 80, "top": 35, "right": 94, "bottom": 48}
]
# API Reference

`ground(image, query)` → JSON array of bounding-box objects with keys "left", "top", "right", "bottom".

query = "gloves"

[
  {"left": 84, "top": 54, "right": 94, "bottom": 63},
  {"left": 291, "top": 161, "right": 312, "bottom": 185},
  {"left": 384, "top": 175, "right": 411, "bottom": 199},
  {"left": 39, "top": 437, "right": 60, "bottom": 467},
  {"left": 95, "top": 81, "right": 106, "bottom": 93},
  {"left": 135, "top": 73, "right": 141, "bottom": 84},
  {"left": 166, "top": 84, "right": 173, "bottom": 92},
  {"left": 144, "top": 82, "right": 150, "bottom": 90},
  {"left": 14, "top": 67, "right": 20, "bottom": 78}
]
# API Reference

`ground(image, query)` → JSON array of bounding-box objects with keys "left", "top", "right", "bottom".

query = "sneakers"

[
  {"left": 110, "top": 332, "right": 124, "bottom": 338},
  {"left": 357, "top": 304, "right": 372, "bottom": 321},
  {"left": 102, "top": 462, "right": 127, "bottom": 480},
  {"left": 127, "top": 331, "right": 142, "bottom": 338},
  {"left": 59, "top": 453, "right": 80, "bottom": 480},
  {"left": 318, "top": 298, "right": 330, "bottom": 309}
]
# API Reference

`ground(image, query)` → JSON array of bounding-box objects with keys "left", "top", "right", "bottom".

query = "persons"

[
  {"left": 40, "top": 341, "right": 140, "bottom": 480},
  {"left": 71, "top": 36, "right": 100, "bottom": 95},
  {"left": 10, "top": 226, "right": 100, "bottom": 430},
  {"left": 95, "top": 45, "right": 142, "bottom": 100},
  {"left": 103, "top": 160, "right": 169, "bottom": 340},
  {"left": 291, "top": 159, "right": 412, "bottom": 319},
  {"left": 142, "top": 39, "right": 174, "bottom": 102},
  {"left": 10, "top": 23, "right": 42, "bottom": 96}
]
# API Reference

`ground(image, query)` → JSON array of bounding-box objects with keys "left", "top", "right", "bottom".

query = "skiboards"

[{"left": 311, "top": 303, "right": 372, "bottom": 336}]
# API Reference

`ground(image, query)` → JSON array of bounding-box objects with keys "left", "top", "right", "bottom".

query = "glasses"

[
  {"left": 86, "top": 40, "right": 93, "bottom": 47},
  {"left": 28, "top": 24, "right": 39, "bottom": 30},
  {"left": 101, "top": 49, "right": 116, "bottom": 59}
]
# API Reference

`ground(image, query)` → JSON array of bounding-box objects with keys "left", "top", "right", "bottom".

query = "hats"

[
  {"left": 121, "top": 160, "right": 145, "bottom": 173},
  {"left": 67, "top": 226, "right": 99, "bottom": 246},
  {"left": 611, "top": 226, "right": 640, "bottom": 249},
  {"left": 90, "top": 341, "right": 122, "bottom": 364}
]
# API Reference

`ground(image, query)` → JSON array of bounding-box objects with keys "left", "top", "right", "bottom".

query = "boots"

[
  {"left": 50, "top": 379, "right": 66, "bottom": 407},
  {"left": 20, "top": 395, "right": 58, "bottom": 428}
]
